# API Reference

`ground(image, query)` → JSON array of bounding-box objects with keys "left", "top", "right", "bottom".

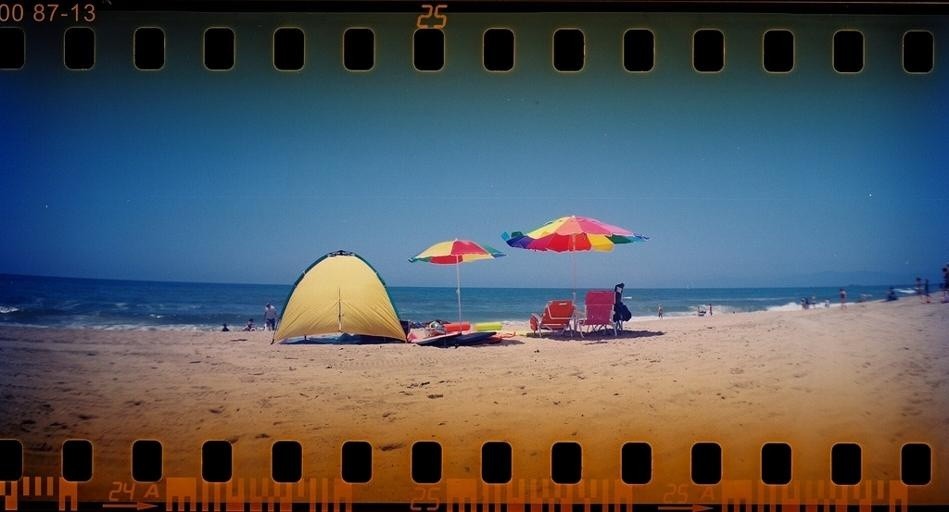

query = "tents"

[{"left": 270, "top": 249, "right": 409, "bottom": 344}]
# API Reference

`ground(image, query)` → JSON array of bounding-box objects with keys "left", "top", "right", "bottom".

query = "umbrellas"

[
  {"left": 503, "top": 214, "right": 650, "bottom": 334},
  {"left": 407, "top": 238, "right": 508, "bottom": 325}
]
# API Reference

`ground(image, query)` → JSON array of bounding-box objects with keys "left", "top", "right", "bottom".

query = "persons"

[
  {"left": 799, "top": 295, "right": 831, "bottom": 311},
  {"left": 916, "top": 263, "right": 949, "bottom": 304},
  {"left": 886, "top": 288, "right": 899, "bottom": 302},
  {"left": 709, "top": 303, "right": 712, "bottom": 316},
  {"left": 264, "top": 303, "right": 277, "bottom": 330},
  {"left": 658, "top": 304, "right": 663, "bottom": 320},
  {"left": 840, "top": 288, "right": 848, "bottom": 310},
  {"left": 243, "top": 318, "right": 254, "bottom": 331},
  {"left": 222, "top": 323, "right": 230, "bottom": 331}
]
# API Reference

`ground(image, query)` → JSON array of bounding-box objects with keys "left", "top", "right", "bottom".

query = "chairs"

[{"left": 529, "top": 290, "right": 619, "bottom": 341}]
